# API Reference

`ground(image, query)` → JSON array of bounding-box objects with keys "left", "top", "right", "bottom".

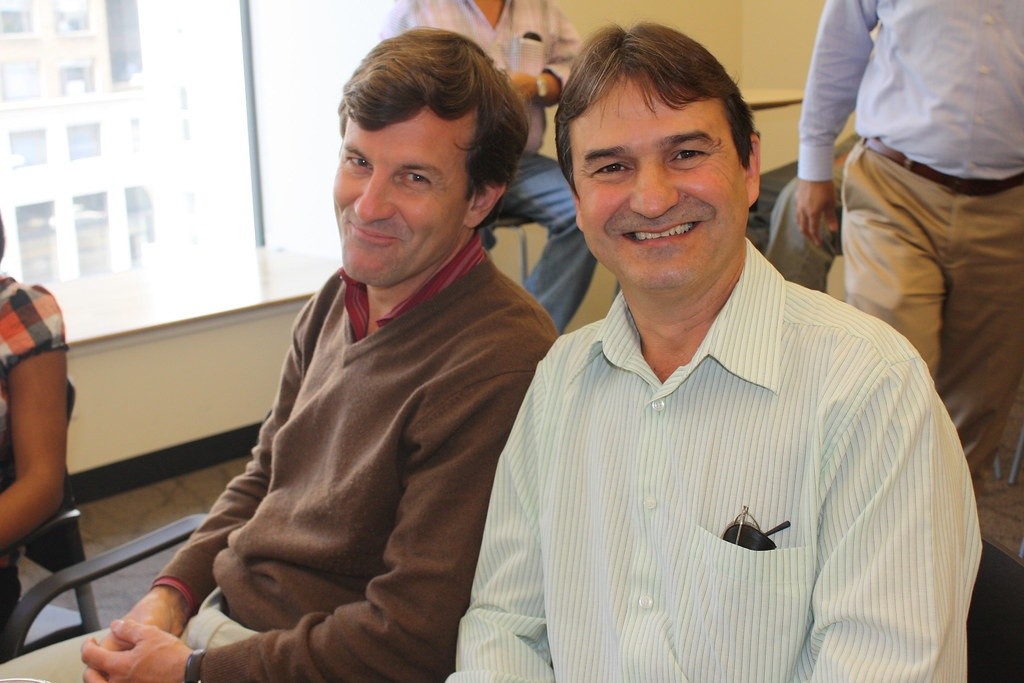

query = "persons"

[
  {"left": 767, "top": 152, "right": 851, "bottom": 292},
  {"left": 382, "top": 0, "right": 597, "bottom": 331},
  {"left": 0, "top": 29, "right": 558, "bottom": 683},
  {"left": 1, "top": 221, "right": 69, "bottom": 623},
  {"left": 447, "top": 24, "right": 983, "bottom": 682},
  {"left": 796, "top": 0, "right": 1024, "bottom": 475}
]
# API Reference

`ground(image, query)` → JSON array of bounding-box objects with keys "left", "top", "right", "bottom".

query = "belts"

[{"left": 865, "top": 138, "right": 1024, "bottom": 196}]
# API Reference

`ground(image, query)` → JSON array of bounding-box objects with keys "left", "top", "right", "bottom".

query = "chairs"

[
  {"left": 0, "top": 376, "right": 103, "bottom": 655},
  {"left": 0, "top": 513, "right": 207, "bottom": 663}
]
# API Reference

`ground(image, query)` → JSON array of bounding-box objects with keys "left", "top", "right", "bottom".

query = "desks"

[{"left": 739, "top": 88, "right": 804, "bottom": 111}]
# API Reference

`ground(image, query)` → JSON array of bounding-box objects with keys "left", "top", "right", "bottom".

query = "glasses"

[{"left": 723, "top": 506, "right": 791, "bottom": 551}]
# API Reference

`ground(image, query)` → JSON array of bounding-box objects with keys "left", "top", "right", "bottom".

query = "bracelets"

[
  {"left": 185, "top": 650, "right": 205, "bottom": 683},
  {"left": 534, "top": 73, "right": 548, "bottom": 100}
]
